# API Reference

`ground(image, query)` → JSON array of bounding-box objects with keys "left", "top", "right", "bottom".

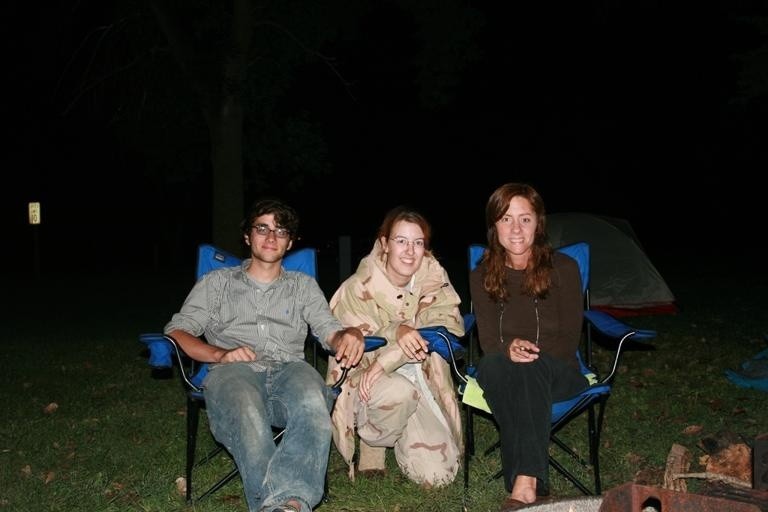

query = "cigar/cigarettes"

[
  {"left": 414, "top": 348, "right": 422, "bottom": 354},
  {"left": 520, "top": 346, "right": 528, "bottom": 351}
]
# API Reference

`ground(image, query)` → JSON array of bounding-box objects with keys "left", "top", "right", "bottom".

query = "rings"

[{"left": 342, "top": 355, "right": 349, "bottom": 359}]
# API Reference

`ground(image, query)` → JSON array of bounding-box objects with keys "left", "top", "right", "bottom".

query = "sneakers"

[{"left": 356, "top": 438, "right": 385, "bottom": 481}]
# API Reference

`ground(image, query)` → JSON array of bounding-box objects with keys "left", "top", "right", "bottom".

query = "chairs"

[
  {"left": 139, "top": 241, "right": 387, "bottom": 507},
  {"left": 454, "top": 234, "right": 658, "bottom": 499}
]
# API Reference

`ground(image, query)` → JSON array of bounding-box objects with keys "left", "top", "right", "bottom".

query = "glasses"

[
  {"left": 249, "top": 225, "right": 291, "bottom": 238},
  {"left": 389, "top": 237, "right": 426, "bottom": 249}
]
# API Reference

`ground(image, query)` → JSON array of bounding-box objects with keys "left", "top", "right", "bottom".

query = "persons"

[
  {"left": 325, "top": 205, "right": 466, "bottom": 490},
  {"left": 163, "top": 199, "right": 365, "bottom": 512},
  {"left": 468, "top": 182, "right": 590, "bottom": 512}
]
